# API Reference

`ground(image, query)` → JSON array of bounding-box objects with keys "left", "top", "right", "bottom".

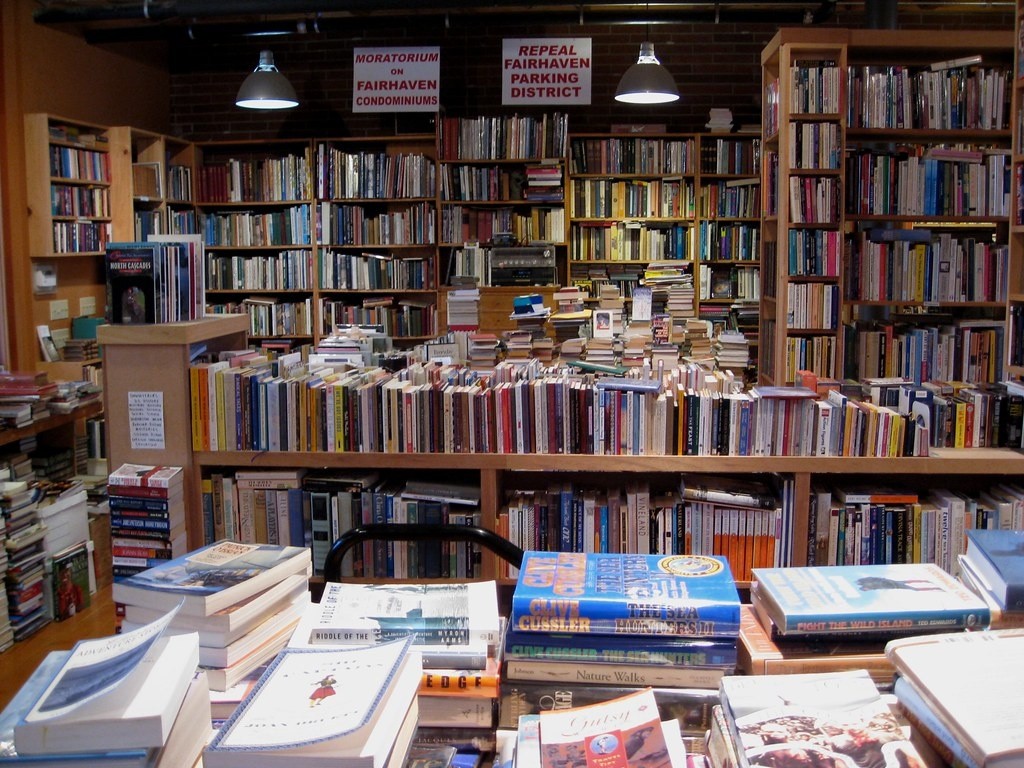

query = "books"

[{"left": 0, "top": 56, "right": 1024, "bottom": 768}]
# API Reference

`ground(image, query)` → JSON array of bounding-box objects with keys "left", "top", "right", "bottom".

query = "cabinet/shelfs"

[{"left": 0, "top": 0, "right": 1023, "bottom": 708}]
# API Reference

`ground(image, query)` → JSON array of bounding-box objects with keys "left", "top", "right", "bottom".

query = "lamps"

[
  {"left": 615, "top": 42, "right": 681, "bottom": 104},
  {"left": 236, "top": 49, "right": 299, "bottom": 108}
]
